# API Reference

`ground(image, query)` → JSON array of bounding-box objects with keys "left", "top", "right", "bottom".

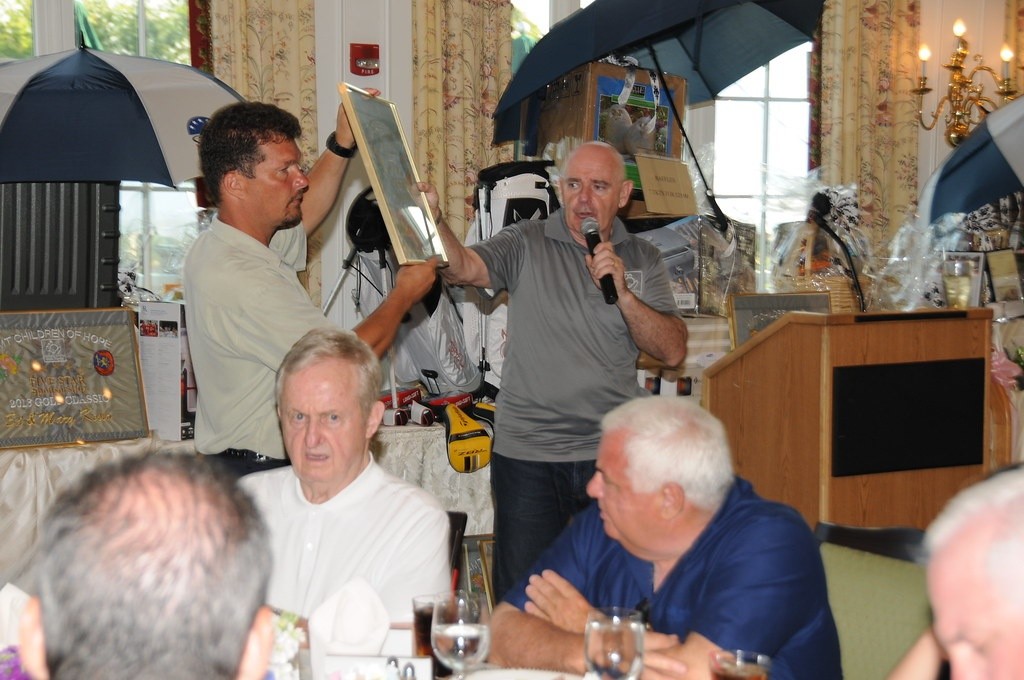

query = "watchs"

[{"left": 325, "top": 131, "right": 357, "bottom": 158}]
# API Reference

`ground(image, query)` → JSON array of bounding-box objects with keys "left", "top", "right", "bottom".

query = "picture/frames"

[
  {"left": 727, "top": 287, "right": 835, "bottom": 350},
  {"left": 1, "top": 307, "right": 150, "bottom": 447},
  {"left": 338, "top": 82, "right": 450, "bottom": 270}
]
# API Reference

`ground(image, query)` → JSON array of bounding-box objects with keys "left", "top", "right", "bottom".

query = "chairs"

[{"left": 818, "top": 542, "right": 933, "bottom": 680}]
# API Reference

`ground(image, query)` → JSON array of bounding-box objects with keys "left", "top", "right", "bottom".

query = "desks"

[{"left": 0, "top": 419, "right": 498, "bottom": 594}]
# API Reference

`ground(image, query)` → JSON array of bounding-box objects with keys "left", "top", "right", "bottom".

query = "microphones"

[{"left": 581, "top": 217, "right": 618, "bottom": 305}]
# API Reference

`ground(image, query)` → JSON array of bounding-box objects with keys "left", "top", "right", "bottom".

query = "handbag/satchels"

[
  {"left": 355, "top": 227, "right": 483, "bottom": 397},
  {"left": 425, "top": 375, "right": 500, "bottom": 473}
]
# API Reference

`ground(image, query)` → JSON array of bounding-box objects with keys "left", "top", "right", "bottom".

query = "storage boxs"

[{"left": 538, "top": 65, "right": 686, "bottom": 162}]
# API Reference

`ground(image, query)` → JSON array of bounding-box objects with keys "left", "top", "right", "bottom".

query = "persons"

[
  {"left": 19, "top": 448, "right": 279, "bottom": 680},
  {"left": 486, "top": 394, "right": 842, "bottom": 679},
  {"left": 183, "top": 87, "right": 442, "bottom": 477},
  {"left": 417, "top": 140, "right": 688, "bottom": 608},
  {"left": 881, "top": 465, "right": 1024, "bottom": 679},
  {"left": 230, "top": 328, "right": 452, "bottom": 658}
]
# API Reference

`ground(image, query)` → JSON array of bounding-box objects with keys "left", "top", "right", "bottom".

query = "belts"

[{"left": 220, "top": 448, "right": 271, "bottom": 462}]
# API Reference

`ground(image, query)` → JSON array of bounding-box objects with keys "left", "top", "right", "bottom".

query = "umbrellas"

[
  {"left": 492, "top": 0, "right": 825, "bottom": 233},
  {"left": 0, "top": 30, "right": 247, "bottom": 192},
  {"left": 913, "top": 95, "right": 1024, "bottom": 231}
]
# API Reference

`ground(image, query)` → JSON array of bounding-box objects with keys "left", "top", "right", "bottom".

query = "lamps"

[{"left": 910, "top": 21, "right": 1019, "bottom": 150}]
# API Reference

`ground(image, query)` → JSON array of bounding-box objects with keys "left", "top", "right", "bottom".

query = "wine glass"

[{"left": 430, "top": 592, "right": 491, "bottom": 679}]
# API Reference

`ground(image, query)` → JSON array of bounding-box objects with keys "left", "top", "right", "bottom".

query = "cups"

[
  {"left": 709, "top": 647, "right": 770, "bottom": 680},
  {"left": 940, "top": 252, "right": 984, "bottom": 310},
  {"left": 583, "top": 609, "right": 645, "bottom": 680},
  {"left": 412, "top": 593, "right": 457, "bottom": 680}
]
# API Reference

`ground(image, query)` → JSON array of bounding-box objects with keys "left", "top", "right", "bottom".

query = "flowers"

[{"left": 990, "top": 340, "right": 1024, "bottom": 391}]
